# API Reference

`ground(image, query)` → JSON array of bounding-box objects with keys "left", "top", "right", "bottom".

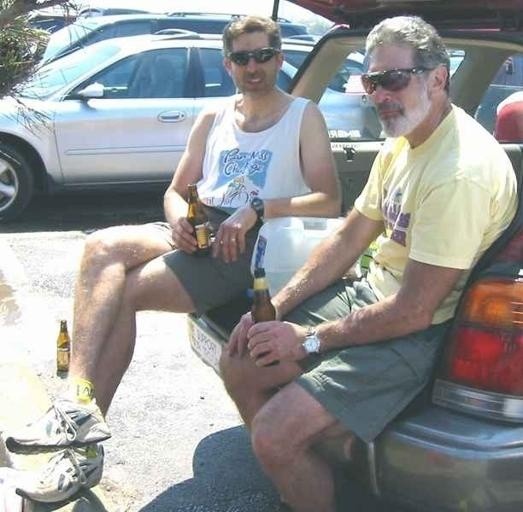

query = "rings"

[{"left": 228, "top": 238, "right": 236, "bottom": 243}]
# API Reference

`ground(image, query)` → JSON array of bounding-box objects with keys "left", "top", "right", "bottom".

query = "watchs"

[
  {"left": 247, "top": 196, "right": 264, "bottom": 230},
  {"left": 299, "top": 324, "right": 322, "bottom": 358}
]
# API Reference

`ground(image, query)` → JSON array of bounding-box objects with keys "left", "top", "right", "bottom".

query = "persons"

[{"left": 5, "top": 14, "right": 343, "bottom": 509}]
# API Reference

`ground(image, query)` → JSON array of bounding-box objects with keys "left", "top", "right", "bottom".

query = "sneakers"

[
  {"left": 13, "top": 440, "right": 106, "bottom": 504},
  {"left": 3, "top": 390, "right": 112, "bottom": 455}
]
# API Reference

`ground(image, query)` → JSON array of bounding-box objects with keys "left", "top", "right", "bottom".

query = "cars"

[
  {"left": 0, "top": 29, "right": 384, "bottom": 228},
  {"left": 13, "top": 0, "right": 338, "bottom": 32},
  {"left": 16, "top": 11, "right": 313, "bottom": 84}
]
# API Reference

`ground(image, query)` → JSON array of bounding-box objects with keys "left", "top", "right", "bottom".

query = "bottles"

[
  {"left": 57, "top": 322, "right": 72, "bottom": 373},
  {"left": 184, "top": 184, "right": 212, "bottom": 255},
  {"left": 252, "top": 268, "right": 280, "bottom": 367}
]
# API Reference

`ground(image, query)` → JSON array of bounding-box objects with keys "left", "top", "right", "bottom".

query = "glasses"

[
  {"left": 224, "top": 47, "right": 280, "bottom": 66},
  {"left": 359, "top": 66, "right": 434, "bottom": 95}
]
